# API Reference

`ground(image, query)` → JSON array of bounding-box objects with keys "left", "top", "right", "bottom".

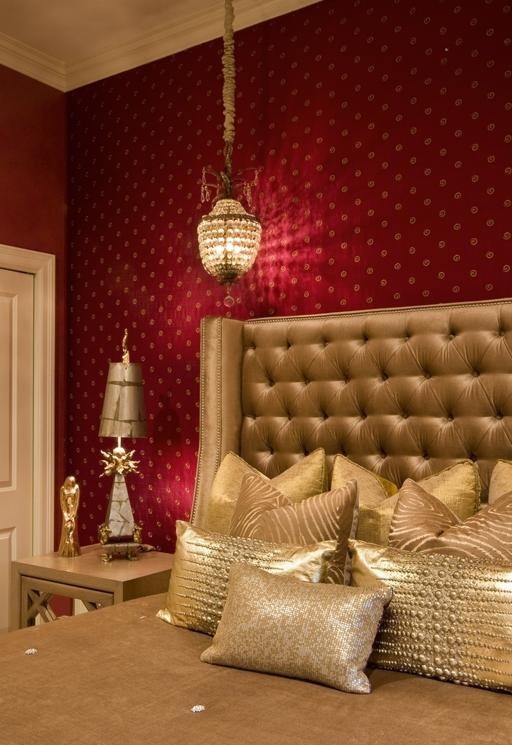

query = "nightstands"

[{"left": 9, "top": 543, "right": 173, "bottom": 630}]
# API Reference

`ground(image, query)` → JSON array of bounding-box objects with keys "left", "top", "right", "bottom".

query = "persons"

[{"left": 56, "top": 475, "right": 82, "bottom": 557}]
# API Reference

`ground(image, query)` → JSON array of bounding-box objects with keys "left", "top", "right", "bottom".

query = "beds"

[{"left": 2, "top": 295, "right": 511, "bottom": 745}]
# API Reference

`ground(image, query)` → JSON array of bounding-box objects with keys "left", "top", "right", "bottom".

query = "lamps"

[
  {"left": 97, "top": 326, "right": 147, "bottom": 563},
  {"left": 196, "top": 0, "right": 262, "bottom": 295}
]
